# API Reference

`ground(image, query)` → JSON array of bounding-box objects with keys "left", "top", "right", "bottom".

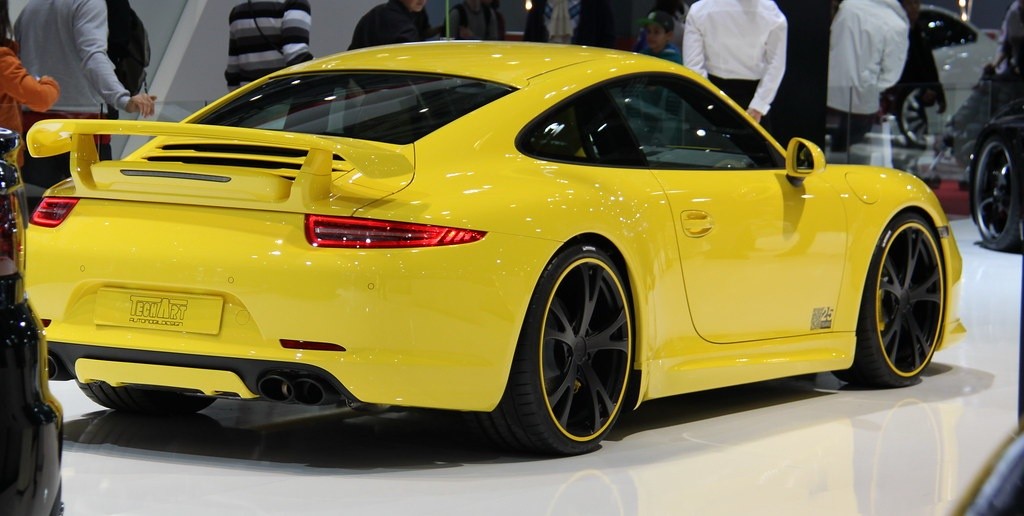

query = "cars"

[
  {"left": 896, "top": 5, "right": 1024, "bottom": 250},
  {"left": 25, "top": 41, "right": 968, "bottom": 453},
  {"left": 0, "top": 127, "right": 64, "bottom": 516}
]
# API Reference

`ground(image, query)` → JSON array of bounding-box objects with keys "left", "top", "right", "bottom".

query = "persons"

[
  {"left": 438, "top": 0, "right": 947, "bottom": 163},
  {"left": 0, "top": 0, "right": 59, "bottom": 168},
  {"left": 224, "top": 0, "right": 317, "bottom": 93},
  {"left": 12, "top": 0, "right": 158, "bottom": 214},
  {"left": 347, "top": 0, "right": 434, "bottom": 50}
]
